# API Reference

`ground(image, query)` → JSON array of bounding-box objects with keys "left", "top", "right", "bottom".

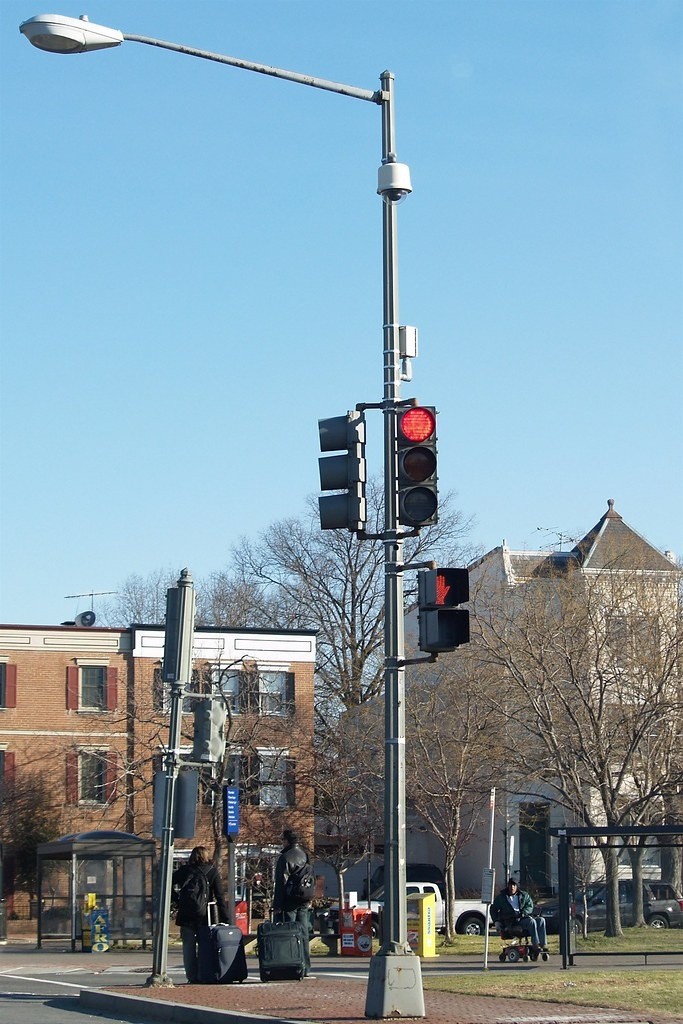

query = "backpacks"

[{"left": 176, "top": 862, "right": 212, "bottom": 915}]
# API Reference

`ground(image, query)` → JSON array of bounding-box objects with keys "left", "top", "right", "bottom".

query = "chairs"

[{"left": 41, "top": 906, "right": 82, "bottom": 939}]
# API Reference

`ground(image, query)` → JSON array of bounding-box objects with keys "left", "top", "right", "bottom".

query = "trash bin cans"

[
  {"left": 316, "top": 917, "right": 340, "bottom": 955},
  {"left": 305, "top": 907, "right": 315, "bottom": 940}
]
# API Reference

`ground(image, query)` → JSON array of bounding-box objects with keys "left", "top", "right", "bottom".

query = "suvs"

[
  {"left": 362, "top": 862, "right": 447, "bottom": 898},
  {"left": 535, "top": 878, "right": 683, "bottom": 933}
]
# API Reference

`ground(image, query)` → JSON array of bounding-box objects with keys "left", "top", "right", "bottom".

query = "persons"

[
  {"left": 273, "top": 829, "right": 311, "bottom": 975},
  {"left": 490, "top": 877, "right": 548, "bottom": 951},
  {"left": 171, "top": 846, "right": 230, "bottom": 984}
]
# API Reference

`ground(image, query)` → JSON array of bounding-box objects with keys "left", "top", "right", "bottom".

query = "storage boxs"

[{"left": 345, "top": 891, "right": 358, "bottom": 909}]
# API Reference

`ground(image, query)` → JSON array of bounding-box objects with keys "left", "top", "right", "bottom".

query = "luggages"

[
  {"left": 257, "top": 908, "right": 305, "bottom": 982},
  {"left": 207, "top": 901, "right": 249, "bottom": 985}
]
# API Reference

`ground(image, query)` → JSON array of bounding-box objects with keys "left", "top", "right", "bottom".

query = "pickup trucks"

[{"left": 330, "top": 880, "right": 495, "bottom": 940}]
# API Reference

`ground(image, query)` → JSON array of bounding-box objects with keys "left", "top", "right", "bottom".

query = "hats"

[{"left": 508, "top": 878, "right": 518, "bottom": 886}]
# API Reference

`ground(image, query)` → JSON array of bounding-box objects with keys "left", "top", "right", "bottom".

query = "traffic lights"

[
  {"left": 396, "top": 407, "right": 441, "bottom": 526},
  {"left": 314, "top": 412, "right": 368, "bottom": 531},
  {"left": 417, "top": 571, "right": 470, "bottom": 656}
]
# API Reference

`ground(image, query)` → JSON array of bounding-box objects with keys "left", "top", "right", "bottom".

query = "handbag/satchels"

[{"left": 286, "top": 852, "right": 313, "bottom": 909}]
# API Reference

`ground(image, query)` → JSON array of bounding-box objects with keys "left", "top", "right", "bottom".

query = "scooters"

[{"left": 490, "top": 905, "right": 552, "bottom": 962}]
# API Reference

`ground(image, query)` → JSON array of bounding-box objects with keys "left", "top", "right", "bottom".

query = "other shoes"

[{"left": 533, "top": 944, "right": 550, "bottom": 952}]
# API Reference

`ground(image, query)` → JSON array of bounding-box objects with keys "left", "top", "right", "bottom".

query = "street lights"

[{"left": 20, "top": 12, "right": 428, "bottom": 1023}]
